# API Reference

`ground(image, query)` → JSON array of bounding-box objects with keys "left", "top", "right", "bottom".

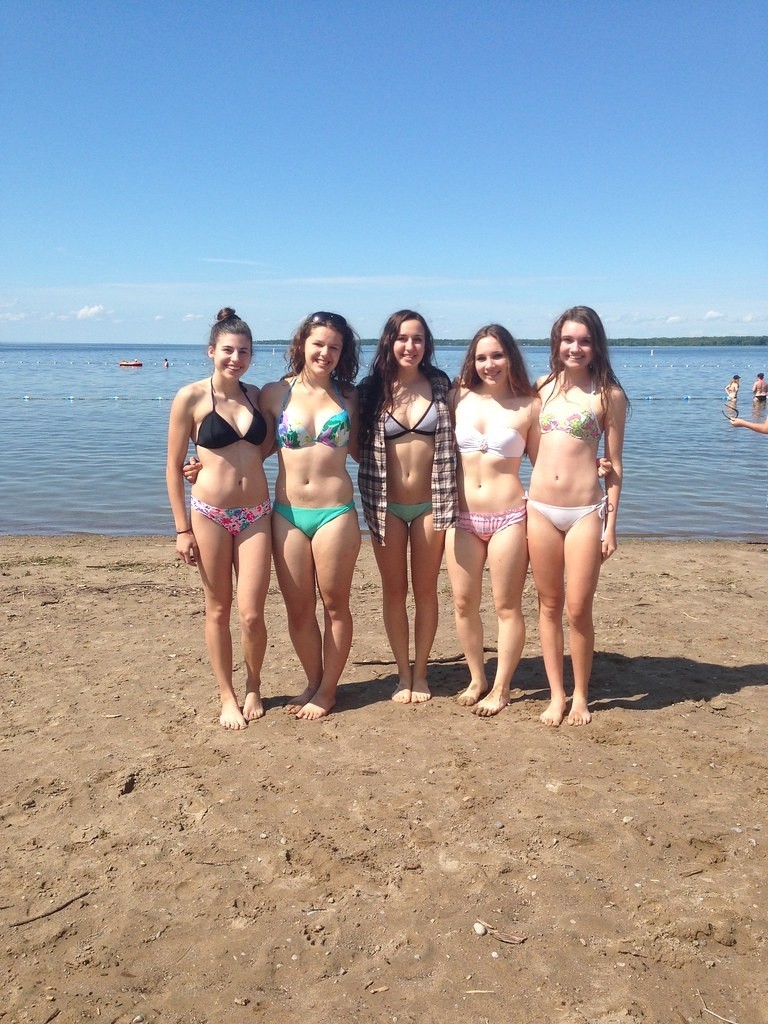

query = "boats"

[{"left": 119, "top": 361, "right": 142, "bottom": 366}]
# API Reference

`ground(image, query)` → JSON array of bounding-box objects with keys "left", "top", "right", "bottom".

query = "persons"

[
  {"left": 447, "top": 324, "right": 613, "bottom": 717},
  {"left": 752, "top": 373, "right": 768, "bottom": 404},
  {"left": 725, "top": 375, "right": 740, "bottom": 402},
  {"left": 167, "top": 308, "right": 273, "bottom": 730},
  {"left": 164, "top": 359, "right": 170, "bottom": 367},
  {"left": 182, "top": 311, "right": 361, "bottom": 720},
  {"left": 730, "top": 415, "right": 768, "bottom": 433},
  {"left": 356, "top": 309, "right": 458, "bottom": 704},
  {"left": 451, "top": 305, "right": 627, "bottom": 727}
]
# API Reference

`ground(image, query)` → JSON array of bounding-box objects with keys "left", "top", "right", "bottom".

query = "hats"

[{"left": 732, "top": 375, "right": 741, "bottom": 379}]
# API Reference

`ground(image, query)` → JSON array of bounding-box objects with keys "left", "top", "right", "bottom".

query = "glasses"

[
  {"left": 721, "top": 403, "right": 739, "bottom": 421},
  {"left": 307, "top": 311, "right": 347, "bottom": 330}
]
